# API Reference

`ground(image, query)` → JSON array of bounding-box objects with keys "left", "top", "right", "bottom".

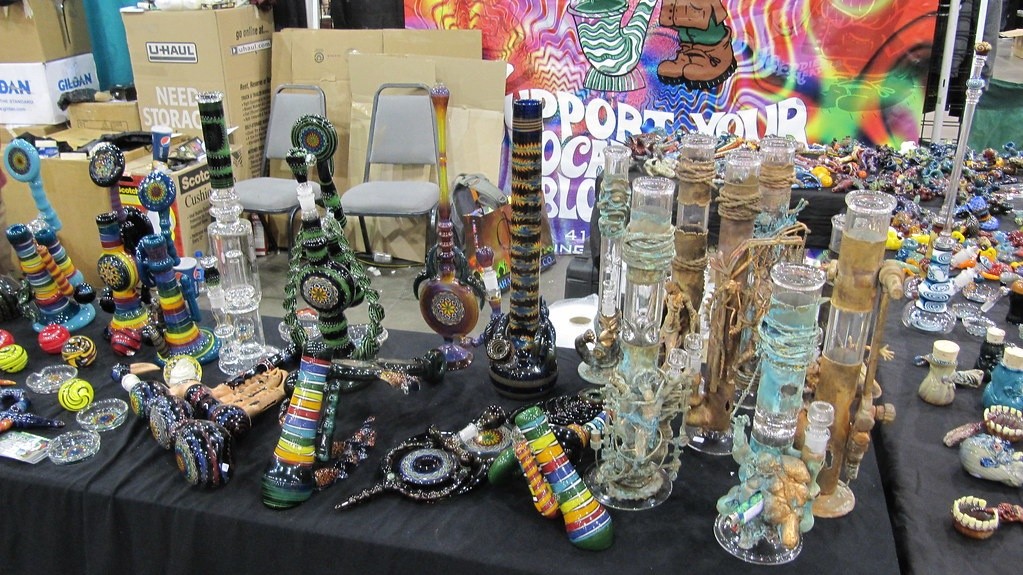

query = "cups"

[{"left": 151, "top": 126, "right": 170, "bottom": 165}]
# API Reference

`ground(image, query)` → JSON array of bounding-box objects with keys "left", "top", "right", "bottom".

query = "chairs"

[
  {"left": 341, "top": 81, "right": 444, "bottom": 276},
  {"left": 210, "top": 85, "right": 328, "bottom": 265}
]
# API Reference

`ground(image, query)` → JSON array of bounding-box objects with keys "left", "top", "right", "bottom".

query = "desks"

[
  {"left": 711, "top": 138, "right": 1023, "bottom": 575},
  {"left": 0, "top": 291, "right": 900, "bottom": 575}
]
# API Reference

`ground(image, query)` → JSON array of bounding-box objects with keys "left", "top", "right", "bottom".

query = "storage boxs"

[{"left": 0, "top": 0, "right": 275, "bottom": 298}]
